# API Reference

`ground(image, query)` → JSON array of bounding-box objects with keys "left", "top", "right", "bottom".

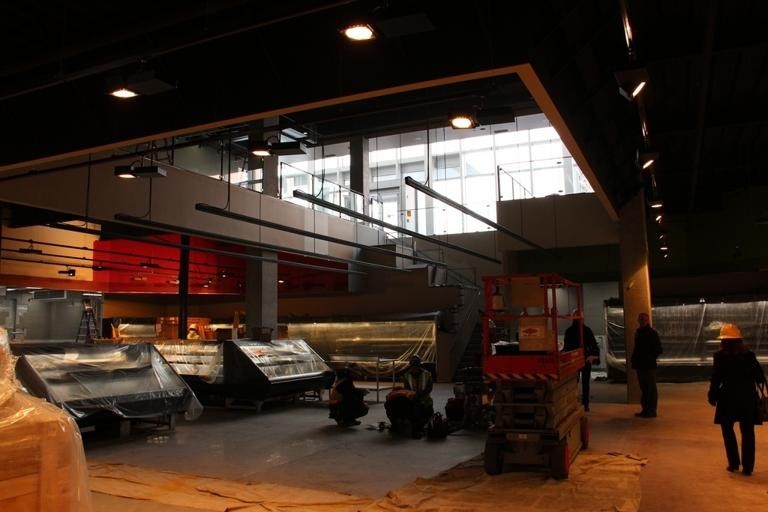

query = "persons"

[
  {"left": 562, "top": 309, "right": 601, "bottom": 412},
  {"left": 707, "top": 323, "right": 766, "bottom": 476},
  {"left": 186, "top": 323, "right": 201, "bottom": 340},
  {"left": 329, "top": 362, "right": 369, "bottom": 428},
  {"left": 404, "top": 356, "right": 433, "bottom": 398},
  {"left": 630, "top": 313, "right": 664, "bottom": 419}
]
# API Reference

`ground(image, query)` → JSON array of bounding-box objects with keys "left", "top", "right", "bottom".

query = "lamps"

[
  {"left": 445, "top": 103, "right": 516, "bottom": 132},
  {"left": 103, "top": 61, "right": 177, "bottom": 102},
  {"left": 335, "top": 1, "right": 438, "bottom": 45},
  {"left": 638, "top": 151, "right": 655, "bottom": 171},
  {"left": 625, "top": 61, "right": 648, "bottom": 100},
  {"left": 648, "top": 186, "right": 663, "bottom": 224},
  {"left": 246, "top": 138, "right": 307, "bottom": 159},
  {"left": 114, "top": 164, "right": 169, "bottom": 182}
]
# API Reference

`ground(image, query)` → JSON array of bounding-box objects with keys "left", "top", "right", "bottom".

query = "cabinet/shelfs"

[
  {"left": 16, "top": 337, "right": 337, "bottom": 450},
  {"left": 286, "top": 319, "right": 439, "bottom": 384},
  {"left": 604, "top": 299, "right": 768, "bottom": 384}
]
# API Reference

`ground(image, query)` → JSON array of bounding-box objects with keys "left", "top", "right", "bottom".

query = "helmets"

[
  {"left": 571, "top": 307, "right": 585, "bottom": 320},
  {"left": 716, "top": 323, "right": 745, "bottom": 340}
]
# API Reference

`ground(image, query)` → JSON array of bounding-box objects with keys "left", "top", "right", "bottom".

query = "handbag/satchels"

[{"left": 759, "top": 396, "right": 768, "bottom": 422}]
